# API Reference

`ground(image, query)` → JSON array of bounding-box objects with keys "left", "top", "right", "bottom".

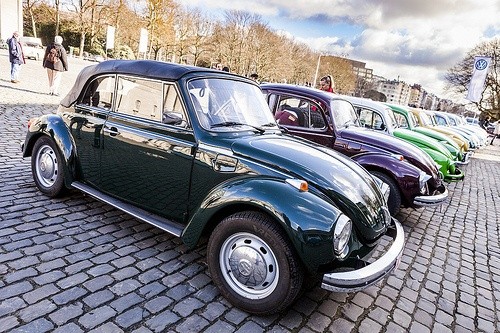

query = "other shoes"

[
  {"left": 50, "top": 92, "right": 58, "bottom": 96},
  {"left": 11, "top": 80, "right": 20, "bottom": 83}
]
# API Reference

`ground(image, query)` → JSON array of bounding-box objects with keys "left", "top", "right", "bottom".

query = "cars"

[
  {"left": 0, "top": 38, "right": 47, "bottom": 61},
  {"left": 21, "top": 61, "right": 405, "bottom": 317},
  {"left": 489, "top": 120, "right": 500, "bottom": 137},
  {"left": 343, "top": 96, "right": 490, "bottom": 184},
  {"left": 212, "top": 81, "right": 449, "bottom": 217}
]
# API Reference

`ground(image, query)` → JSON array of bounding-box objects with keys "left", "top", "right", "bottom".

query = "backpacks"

[{"left": 46, "top": 44, "right": 61, "bottom": 63}]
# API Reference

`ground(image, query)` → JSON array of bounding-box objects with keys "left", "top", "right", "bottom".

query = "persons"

[
  {"left": 8, "top": 31, "right": 25, "bottom": 83},
  {"left": 312, "top": 76, "right": 334, "bottom": 113},
  {"left": 223, "top": 67, "right": 229, "bottom": 72},
  {"left": 490, "top": 121, "right": 500, "bottom": 145},
  {"left": 480, "top": 116, "right": 490, "bottom": 130},
  {"left": 251, "top": 74, "right": 259, "bottom": 82},
  {"left": 43, "top": 36, "right": 69, "bottom": 96},
  {"left": 276, "top": 107, "right": 329, "bottom": 128}
]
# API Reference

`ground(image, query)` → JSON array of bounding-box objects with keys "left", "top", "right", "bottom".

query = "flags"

[{"left": 467, "top": 56, "right": 491, "bottom": 102}]
